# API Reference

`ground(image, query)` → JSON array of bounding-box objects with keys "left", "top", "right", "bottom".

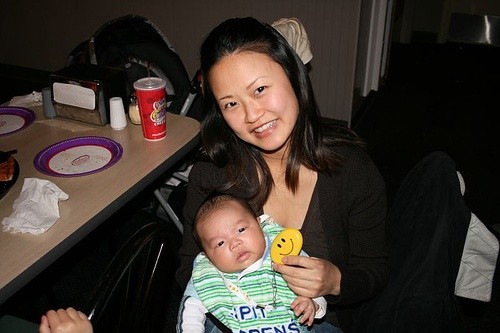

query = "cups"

[{"left": 133, "top": 77, "right": 167, "bottom": 143}]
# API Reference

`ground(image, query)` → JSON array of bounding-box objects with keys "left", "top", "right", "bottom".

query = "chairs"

[{"left": 358, "top": 151, "right": 457, "bottom": 332}]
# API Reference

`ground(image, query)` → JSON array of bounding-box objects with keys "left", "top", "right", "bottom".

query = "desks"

[{"left": 0, "top": 89, "right": 203, "bottom": 305}]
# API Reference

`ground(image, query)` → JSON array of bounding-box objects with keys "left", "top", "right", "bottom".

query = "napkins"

[{"left": 2, "top": 177, "right": 69, "bottom": 236}]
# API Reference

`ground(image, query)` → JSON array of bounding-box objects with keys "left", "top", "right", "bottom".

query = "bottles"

[
  {"left": 42, "top": 88, "right": 57, "bottom": 119},
  {"left": 129, "top": 95, "right": 142, "bottom": 126},
  {"left": 109, "top": 97, "right": 127, "bottom": 130}
]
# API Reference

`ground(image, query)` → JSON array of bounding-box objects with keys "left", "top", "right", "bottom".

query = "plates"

[
  {"left": 0, "top": 150, "right": 20, "bottom": 199},
  {"left": 0, "top": 106, "right": 36, "bottom": 138},
  {"left": 32, "top": 136, "right": 123, "bottom": 179}
]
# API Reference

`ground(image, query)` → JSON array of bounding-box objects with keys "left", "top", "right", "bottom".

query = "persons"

[
  {"left": 39, "top": 308, "right": 93, "bottom": 333},
  {"left": 176, "top": 195, "right": 327, "bottom": 333},
  {"left": 165, "top": 18, "right": 390, "bottom": 333}
]
